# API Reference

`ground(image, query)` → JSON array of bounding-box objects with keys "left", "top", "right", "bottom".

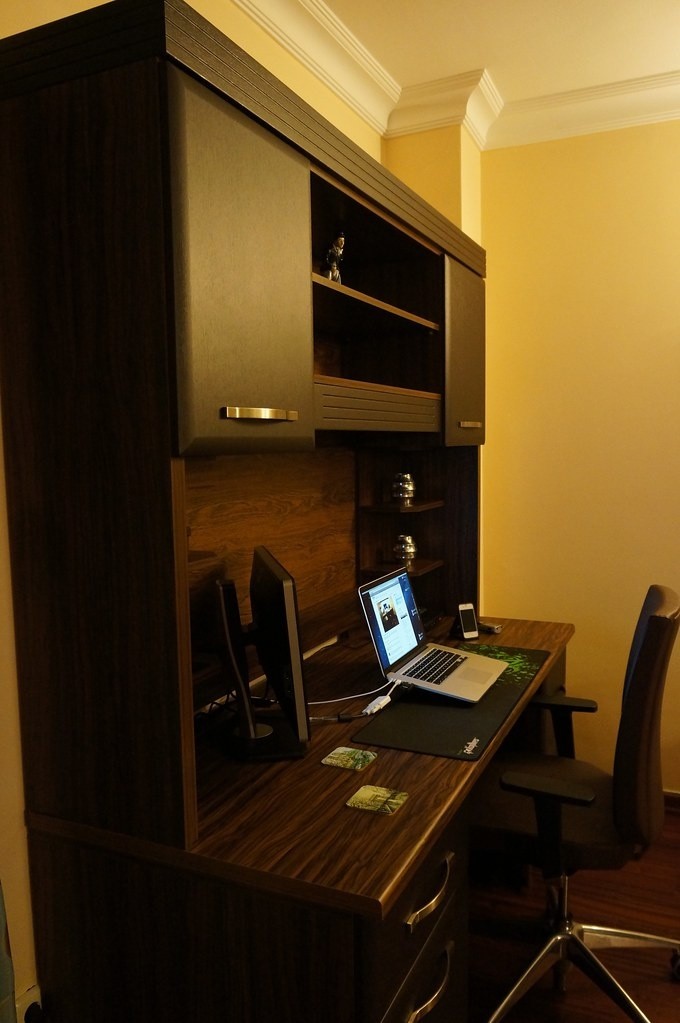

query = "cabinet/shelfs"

[{"left": 0, "top": 0, "right": 488, "bottom": 847}]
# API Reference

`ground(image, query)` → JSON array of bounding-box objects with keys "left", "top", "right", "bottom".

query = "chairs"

[{"left": 473, "top": 578, "right": 680, "bottom": 1023}]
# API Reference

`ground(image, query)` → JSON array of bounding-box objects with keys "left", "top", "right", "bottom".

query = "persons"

[{"left": 319, "top": 232, "right": 345, "bottom": 285}]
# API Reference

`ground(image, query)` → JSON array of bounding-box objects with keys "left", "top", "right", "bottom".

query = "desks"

[{"left": 30, "top": 605, "right": 576, "bottom": 1023}]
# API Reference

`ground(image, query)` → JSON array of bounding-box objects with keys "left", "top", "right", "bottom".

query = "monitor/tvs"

[{"left": 219, "top": 546, "right": 312, "bottom": 762}]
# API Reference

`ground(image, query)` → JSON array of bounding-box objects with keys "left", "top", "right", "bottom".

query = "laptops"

[{"left": 357, "top": 566, "right": 509, "bottom": 703}]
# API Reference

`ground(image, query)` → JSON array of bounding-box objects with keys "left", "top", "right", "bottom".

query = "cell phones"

[{"left": 458, "top": 603, "right": 479, "bottom": 639}]
